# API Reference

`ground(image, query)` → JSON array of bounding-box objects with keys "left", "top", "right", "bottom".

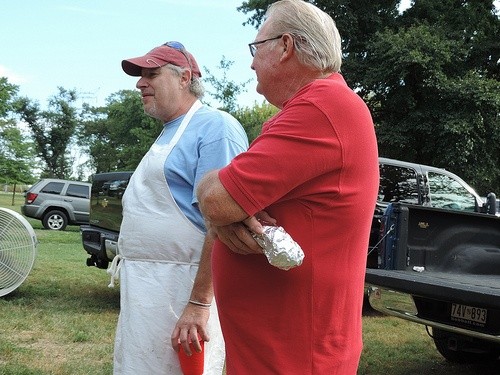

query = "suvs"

[{"left": 21, "top": 178, "right": 92, "bottom": 232}]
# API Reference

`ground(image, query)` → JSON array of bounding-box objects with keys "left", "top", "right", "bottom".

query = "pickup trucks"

[
  {"left": 81, "top": 156, "right": 500, "bottom": 269},
  {"left": 366, "top": 201, "right": 500, "bottom": 363}
]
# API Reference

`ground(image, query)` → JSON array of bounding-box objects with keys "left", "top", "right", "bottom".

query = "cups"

[{"left": 176, "top": 333, "right": 203, "bottom": 375}]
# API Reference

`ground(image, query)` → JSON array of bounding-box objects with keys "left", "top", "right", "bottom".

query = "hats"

[{"left": 121, "top": 44, "right": 202, "bottom": 77}]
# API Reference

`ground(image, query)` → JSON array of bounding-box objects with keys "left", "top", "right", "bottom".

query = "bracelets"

[{"left": 189, "top": 299, "right": 211, "bottom": 307}]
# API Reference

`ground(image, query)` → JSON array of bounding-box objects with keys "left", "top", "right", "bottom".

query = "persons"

[
  {"left": 117, "top": 41, "right": 249, "bottom": 375},
  {"left": 197, "top": 0, "right": 380, "bottom": 375}
]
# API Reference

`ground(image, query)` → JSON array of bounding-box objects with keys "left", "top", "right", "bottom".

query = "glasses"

[
  {"left": 161, "top": 41, "right": 192, "bottom": 69},
  {"left": 248, "top": 35, "right": 282, "bottom": 57}
]
